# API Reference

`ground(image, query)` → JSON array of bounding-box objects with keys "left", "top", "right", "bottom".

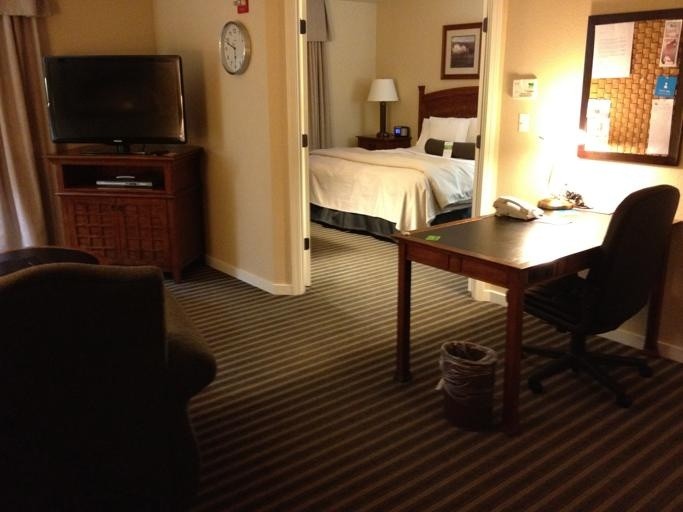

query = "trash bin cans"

[{"left": 439, "top": 340, "right": 499, "bottom": 435}]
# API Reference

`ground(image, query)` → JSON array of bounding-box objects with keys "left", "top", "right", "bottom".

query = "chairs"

[{"left": 506, "top": 184, "right": 681, "bottom": 409}]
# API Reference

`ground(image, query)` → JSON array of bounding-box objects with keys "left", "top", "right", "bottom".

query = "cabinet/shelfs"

[{"left": 42, "top": 147, "right": 204, "bottom": 284}]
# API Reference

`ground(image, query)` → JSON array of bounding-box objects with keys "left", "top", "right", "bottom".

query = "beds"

[{"left": 309, "top": 85, "right": 479, "bottom": 243}]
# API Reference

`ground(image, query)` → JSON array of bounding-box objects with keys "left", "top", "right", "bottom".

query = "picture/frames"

[
  {"left": 577, "top": 8, "right": 683, "bottom": 167},
  {"left": 440, "top": 22, "right": 483, "bottom": 80}
]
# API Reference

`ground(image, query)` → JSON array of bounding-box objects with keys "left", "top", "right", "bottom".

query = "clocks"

[{"left": 219, "top": 20, "right": 251, "bottom": 76}]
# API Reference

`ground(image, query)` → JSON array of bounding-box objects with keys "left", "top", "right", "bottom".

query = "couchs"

[{"left": 0, "top": 246, "right": 216, "bottom": 512}]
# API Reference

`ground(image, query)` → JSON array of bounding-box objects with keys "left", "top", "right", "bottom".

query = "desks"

[{"left": 389, "top": 207, "right": 683, "bottom": 437}]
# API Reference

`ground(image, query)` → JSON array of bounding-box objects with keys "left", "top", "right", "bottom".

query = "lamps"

[{"left": 366, "top": 78, "right": 400, "bottom": 138}]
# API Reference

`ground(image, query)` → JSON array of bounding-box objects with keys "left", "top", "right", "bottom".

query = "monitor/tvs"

[{"left": 42, "top": 54, "right": 186, "bottom": 156}]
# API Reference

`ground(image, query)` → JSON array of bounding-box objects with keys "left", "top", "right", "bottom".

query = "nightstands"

[{"left": 355, "top": 135, "right": 413, "bottom": 151}]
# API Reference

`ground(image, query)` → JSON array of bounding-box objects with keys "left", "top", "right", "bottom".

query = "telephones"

[{"left": 493, "top": 195, "right": 545, "bottom": 221}]
